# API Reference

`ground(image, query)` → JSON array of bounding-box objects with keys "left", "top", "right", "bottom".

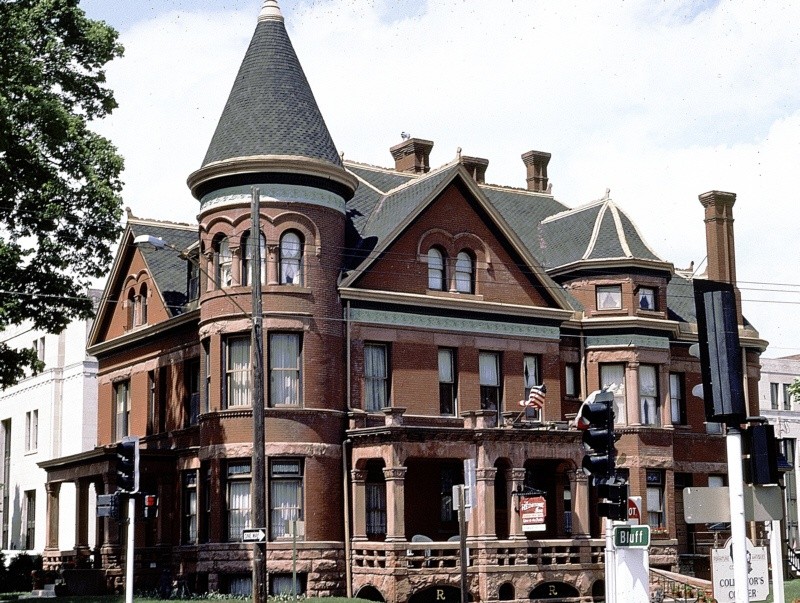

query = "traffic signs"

[{"left": 241, "top": 528, "right": 266, "bottom": 544}]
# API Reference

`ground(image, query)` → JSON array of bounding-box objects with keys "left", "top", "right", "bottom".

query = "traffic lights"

[
  {"left": 688, "top": 344, "right": 704, "bottom": 401},
  {"left": 142, "top": 492, "right": 160, "bottom": 520},
  {"left": 581, "top": 401, "right": 615, "bottom": 477},
  {"left": 101, "top": 441, "right": 139, "bottom": 494}
]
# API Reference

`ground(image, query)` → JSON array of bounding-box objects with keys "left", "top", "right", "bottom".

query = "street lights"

[{"left": 133, "top": 234, "right": 268, "bottom": 603}]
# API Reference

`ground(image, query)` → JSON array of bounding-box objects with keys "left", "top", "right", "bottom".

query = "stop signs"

[{"left": 612, "top": 496, "right": 642, "bottom": 528}]
